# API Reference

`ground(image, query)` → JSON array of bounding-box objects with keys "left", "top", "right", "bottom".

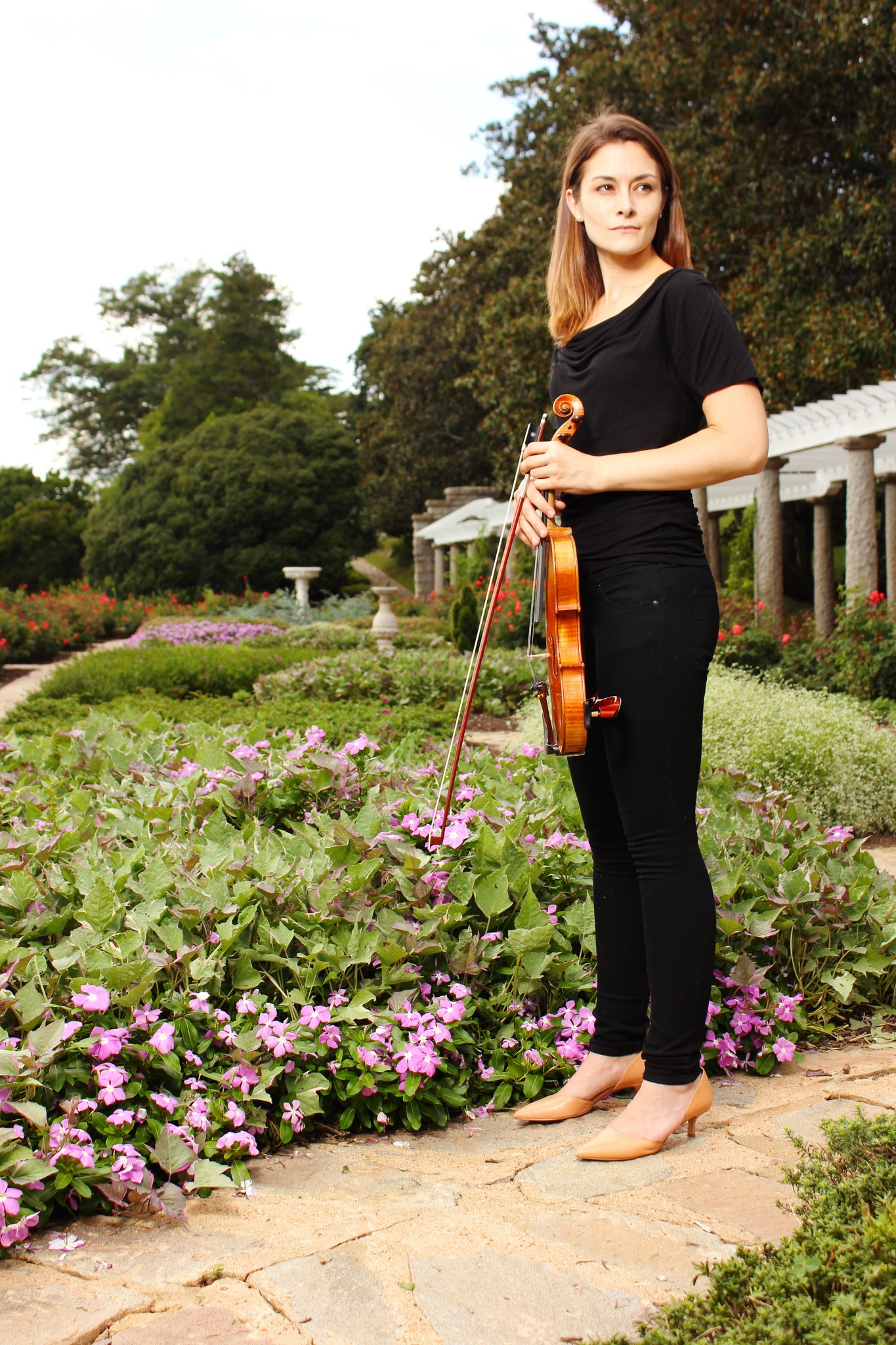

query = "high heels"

[
  {"left": 514, "top": 1052, "right": 644, "bottom": 1121},
  {"left": 577, "top": 1068, "right": 711, "bottom": 1159}
]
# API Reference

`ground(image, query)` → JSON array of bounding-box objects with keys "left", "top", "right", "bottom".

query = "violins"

[{"left": 527, "top": 393, "right": 593, "bottom": 756}]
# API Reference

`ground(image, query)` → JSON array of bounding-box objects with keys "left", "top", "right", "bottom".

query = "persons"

[{"left": 512, "top": 110, "right": 769, "bottom": 1159}]
